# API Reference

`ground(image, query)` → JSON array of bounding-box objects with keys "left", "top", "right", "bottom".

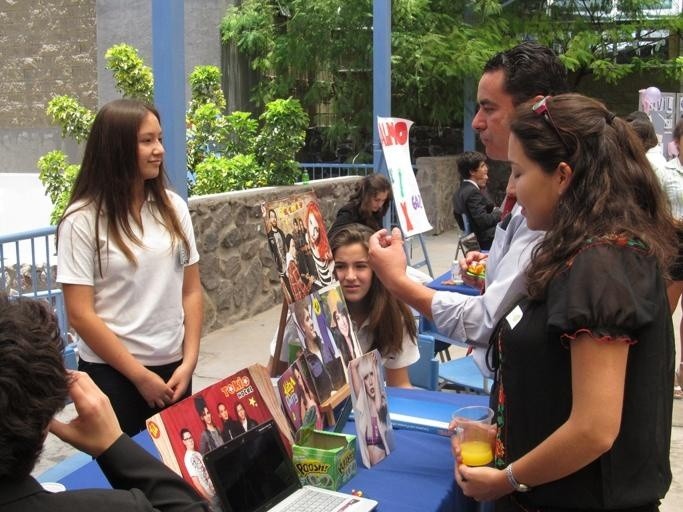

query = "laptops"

[{"left": 202, "top": 418, "right": 379, "bottom": 511}]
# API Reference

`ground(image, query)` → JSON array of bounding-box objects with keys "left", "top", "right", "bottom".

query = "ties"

[{"left": 498, "top": 194, "right": 517, "bottom": 223}]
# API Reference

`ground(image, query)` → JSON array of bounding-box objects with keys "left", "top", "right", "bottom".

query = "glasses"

[{"left": 531, "top": 95, "right": 576, "bottom": 160}]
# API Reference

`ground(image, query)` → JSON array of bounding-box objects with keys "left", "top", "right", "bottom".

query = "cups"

[{"left": 452, "top": 406, "right": 494, "bottom": 468}]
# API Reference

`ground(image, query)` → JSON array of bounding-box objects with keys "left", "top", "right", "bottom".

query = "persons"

[
  {"left": 325, "top": 173, "right": 434, "bottom": 316},
  {"left": 215, "top": 403, "right": 245, "bottom": 444},
  {"left": 178, "top": 428, "right": 222, "bottom": 510},
  {"left": 264, "top": 224, "right": 421, "bottom": 390},
  {"left": 433, "top": 92, "right": 677, "bottom": 512},
  {"left": 303, "top": 200, "right": 336, "bottom": 285},
  {"left": 56, "top": 98, "right": 203, "bottom": 439},
  {"left": 347, "top": 348, "right": 390, "bottom": 468},
  {"left": 264, "top": 209, "right": 290, "bottom": 274},
  {"left": 284, "top": 233, "right": 315, "bottom": 303},
  {"left": 193, "top": 394, "right": 227, "bottom": 452},
  {"left": 367, "top": 42, "right": 571, "bottom": 348},
  {"left": 655, "top": 119, "right": 683, "bottom": 399},
  {"left": 297, "top": 218, "right": 309, "bottom": 246},
  {"left": 292, "top": 218, "right": 301, "bottom": 247},
  {"left": 0, "top": 292, "right": 213, "bottom": 511},
  {"left": 633, "top": 116, "right": 668, "bottom": 171},
  {"left": 459, "top": 162, "right": 488, "bottom": 194},
  {"left": 325, "top": 287, "right": 363, "bottom": 384},
  {"left": 289, "top": 361, "right": 325, "bottom": 430},
  {"left": 233, "top": 402, "right": 259, "bottom": 436},
  {"left": 451, "top": 150, "right": 504, "bottom": 251},
  {"left": 627, "top": 110, "right": 650, "bottom": 123},
  {"left": 292, "top": 301, "right": 347, "bottom": 404}
]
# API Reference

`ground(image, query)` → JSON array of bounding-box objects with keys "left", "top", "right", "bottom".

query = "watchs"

[{"left": 506, "top": 463, "right": 530, "bottom": 493}]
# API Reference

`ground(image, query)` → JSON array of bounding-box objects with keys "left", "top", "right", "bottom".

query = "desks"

[
  {"left": 35, "top": 386, "right": 490, "bottom": 512},
  {"left": 426, "top": 249, "right": 489, "bottom": 296}
]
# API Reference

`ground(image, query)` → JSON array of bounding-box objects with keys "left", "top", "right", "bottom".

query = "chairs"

[
  {"left": 408, "top": 334, "right": 439, "bottom": 392},
  {"left": 419, "top": 313, "right": 494, "bottom": 394}
]
